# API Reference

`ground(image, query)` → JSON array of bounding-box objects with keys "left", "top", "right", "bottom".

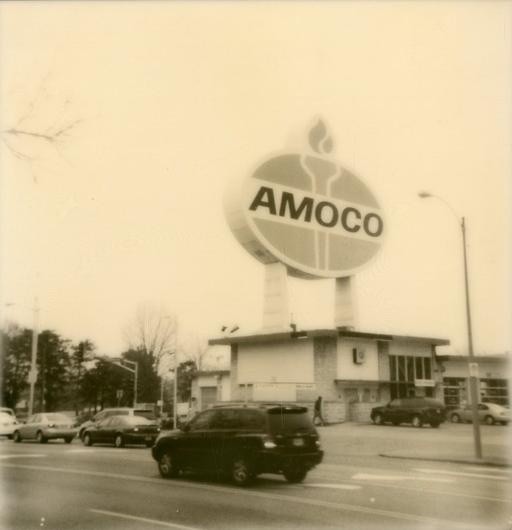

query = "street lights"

[{"left": 416, "top": 185, "right": 485, "bottom": 463}]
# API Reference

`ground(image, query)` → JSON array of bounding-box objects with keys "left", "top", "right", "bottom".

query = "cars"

[
  {"left": 150, "top": 399, "right": 325, "bottom": 485},
  {"left": 12, "top": 412, "right": 76, "bottom": 443},
  {"left": 447, "top": 401, "right": 512, "bottom": 425},
  {"left": 370, "top": 395, "right": 449, "bottom": 428},
  {"left": 0, "top": 406, "right": 18, "bottom": 440},
  {"left": 77, "top": 407, "right": 160, "bottom": 447}
]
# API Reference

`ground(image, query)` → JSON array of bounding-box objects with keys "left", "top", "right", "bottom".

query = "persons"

[{"left": 310, "top": 395, "right": 330, "bottom": 427}]
